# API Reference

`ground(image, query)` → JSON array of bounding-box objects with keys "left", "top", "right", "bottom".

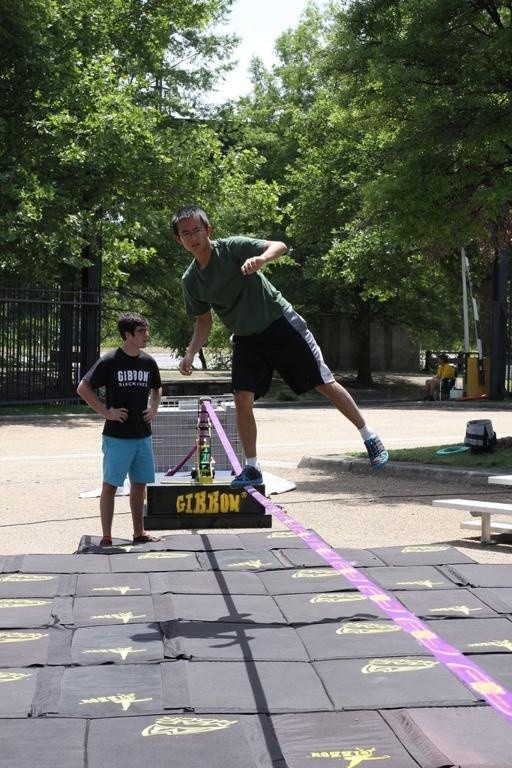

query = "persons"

[
  {"left": 423, "top": 355, "right": 454, "bottom": 401},
  {"left": 172, "top": 207, "right": 388, "bottom": 490},
  {"left": 76, "top": 311, "right": 163, "bottom": 547}
]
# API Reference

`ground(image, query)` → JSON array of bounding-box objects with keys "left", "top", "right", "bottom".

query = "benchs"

[{"left": 431, "top": 498, "right": 511, "bottom": 544}]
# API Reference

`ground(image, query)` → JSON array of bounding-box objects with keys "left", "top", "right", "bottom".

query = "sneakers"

[
  {"left": 230, "top": 465, "right": 262, "bottom": 488},
  {"left": 99, "top": 536, "right": 112, "bottom": 546},
  {"left": 133, "top": 533, "right": 161, "bottom": 542},
  {"left": 363, "top": 432, "right": 389, "bottom": 466}
]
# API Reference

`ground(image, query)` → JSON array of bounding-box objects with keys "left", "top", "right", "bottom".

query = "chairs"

[{"left": 432, "top": 377, "right": 456, "bottom": 401}]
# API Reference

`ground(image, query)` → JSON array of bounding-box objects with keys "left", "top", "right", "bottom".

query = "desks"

[{"left": 486, "top": 473, "right": 512, "bottom": 486}]
